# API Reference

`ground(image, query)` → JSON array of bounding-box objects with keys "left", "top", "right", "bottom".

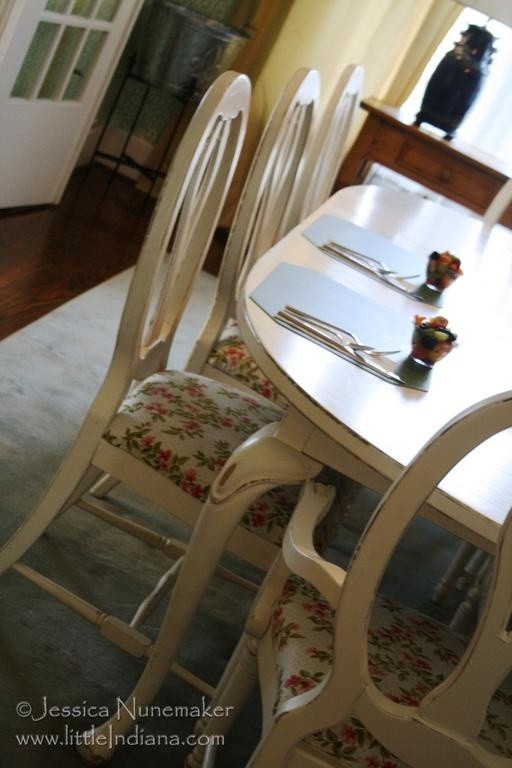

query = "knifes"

[
  {"left": 278, "top": 310, "right": 407, "bottom": 384},
  {"left": 318, "top": 246, "right": 426, "bottom": 301}
]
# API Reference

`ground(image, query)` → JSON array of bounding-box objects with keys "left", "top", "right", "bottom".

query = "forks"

[
  {"left": 284, "top": 304, "right": 402, "bottom": 356},
  {"left": 322, "top": 243, "right": 424, "bottom": 283}
]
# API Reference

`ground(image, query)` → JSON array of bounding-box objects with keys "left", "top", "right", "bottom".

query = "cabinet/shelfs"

[{"left": 333, "top": 98, "right": 510, "bottom": 228}]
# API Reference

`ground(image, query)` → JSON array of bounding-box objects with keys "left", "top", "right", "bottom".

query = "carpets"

[{"left": 1, "top": 259, "right": 512, "bottom": 767}]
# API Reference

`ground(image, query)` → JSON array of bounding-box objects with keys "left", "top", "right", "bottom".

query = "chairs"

[
  {"left": 297, "top": 60, "right": 370, "bottom": 223},
  {"left": 1, "top": 72, "right": 330, "bottom": 734},
  {"left": 183, "top": 387, "right": 510, "bottom": 766},
  {"left": 482, "top": 181, "right": 510, "bottom": 226},
  {"left": 88, "top": 71, "right": 361, "bottom": 595}
]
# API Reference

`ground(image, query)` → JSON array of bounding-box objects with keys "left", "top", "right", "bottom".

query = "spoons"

[
  {"left": 327, "top": 237, "right": 401, "bottom": 280},
  {"left": 282, "top": 309, "right": 376, "bottom": 354}
]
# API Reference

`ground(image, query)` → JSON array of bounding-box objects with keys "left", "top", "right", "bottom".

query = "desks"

[{"left": 74, "top": 49, "right": 202, "bottom": 236}]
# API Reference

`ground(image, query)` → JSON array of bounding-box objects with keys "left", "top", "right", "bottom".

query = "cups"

[
  {"left": 409, "top": 319, "right": 458, "bottom": 369},
  {"left": 424, "top": 253, "right": 463, "bottom": 292}
]
